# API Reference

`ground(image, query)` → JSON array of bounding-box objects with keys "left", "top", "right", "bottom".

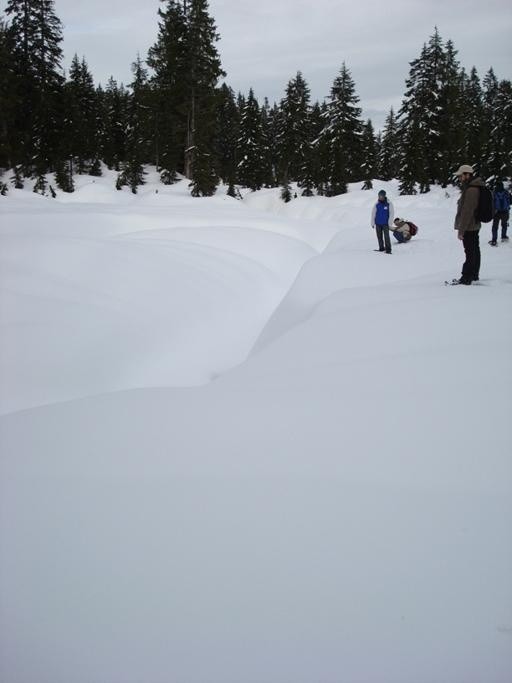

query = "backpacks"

[
  {"left": 465, "top": 184, "right": 496, "bottom": 222},
  {"left": 495, "top": 190, "right": 509, "bottom": 211},
  {"left": 404, "top": 221, "right": 418, "bottom": 234}
]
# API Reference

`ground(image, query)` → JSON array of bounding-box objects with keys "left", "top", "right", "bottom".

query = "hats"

[
  {"left": 452, "top": 164, "right": 475, "bottom": 175},
  {"left": 378, "top": 189, "right": 386, "bottom": 197}
]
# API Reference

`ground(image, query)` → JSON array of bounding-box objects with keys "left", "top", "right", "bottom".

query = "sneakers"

[
  {"left": 386, "top": 250, "right": 392, "bottom": 254},
  {"left": 459, "top": 278, "right": 471, "bottom": 284},
  {"left": 502, "top": 235, "right": 508, "bottom": 239},
  {"left": 488, "top": 240, "right": 496, "bottom": 245}
]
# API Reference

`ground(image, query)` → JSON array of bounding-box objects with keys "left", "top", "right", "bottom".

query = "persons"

[
  {"left": 389, "top": 218, "right": 411, "bottom": 243},
  {"left": 452, "top": 165, "right": 485, "bottom": 285},
  {"left": 371, "top": 190, "right": 394, "bottom": 255},
  {"left": 488, "top": 182, "right": 512, "bottom": 246}
]
